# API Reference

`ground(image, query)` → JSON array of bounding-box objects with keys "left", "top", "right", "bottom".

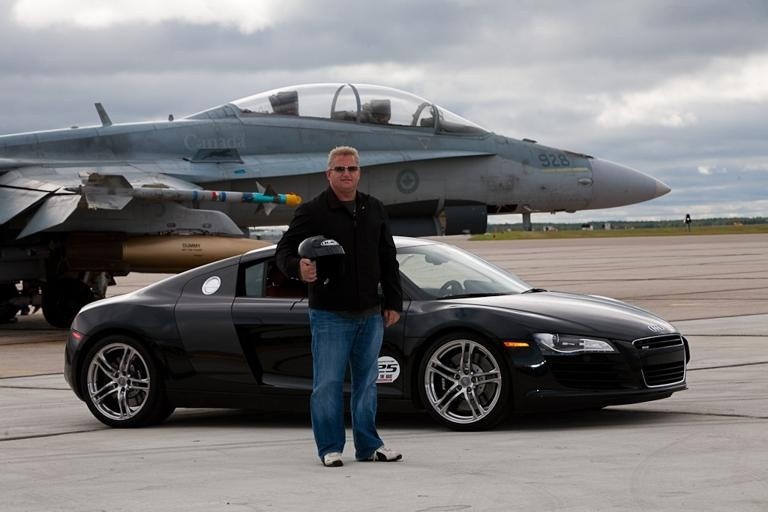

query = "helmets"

[{"left": 297, "top": 235, "right": 345, "bottom": 258}]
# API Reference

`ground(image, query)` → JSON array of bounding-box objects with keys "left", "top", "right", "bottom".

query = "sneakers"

[
  {"left": 356, "top": 445, "right": 402, "bottom": 461},
  {"left": 323, "top": 452, "right": 344, "bottom": 466}
]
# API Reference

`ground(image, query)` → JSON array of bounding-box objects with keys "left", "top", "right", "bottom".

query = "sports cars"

[{"left": 62, "top": 233, "right": 693, "bottom": 434}]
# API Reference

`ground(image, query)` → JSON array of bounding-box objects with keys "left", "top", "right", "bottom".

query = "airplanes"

[{"left": 0, "top": 79, "right": 675, "bottom": 332}]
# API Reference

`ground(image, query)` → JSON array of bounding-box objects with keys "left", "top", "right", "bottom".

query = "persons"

[
  {"left": 274, "top": 146, "right": 403, "bottom": 467},
  {"left": 684, "top": 213, "right": 692, "bottom": 232}
]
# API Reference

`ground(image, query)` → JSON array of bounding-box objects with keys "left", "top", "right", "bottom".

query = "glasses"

[{"left": 330, "top": 166, "right": 359, "bottom": 172}]
